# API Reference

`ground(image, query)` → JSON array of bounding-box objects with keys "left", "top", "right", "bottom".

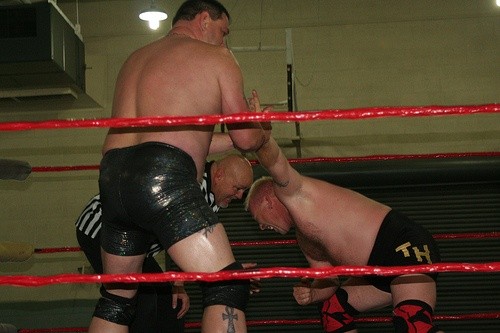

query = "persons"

[
  {"left": 244, "top": 89, "right": 444, "bottom": 333},
  {"left": 75, "top": 154, "right": 260, "bottom": 333},
  {"left": 89, "top": 0, "right": 266, "bottom": 333}
]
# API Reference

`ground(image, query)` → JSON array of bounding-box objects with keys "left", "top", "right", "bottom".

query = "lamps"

[{"left": 138, "top": 0, "right": 168, "bottom": 31}]
